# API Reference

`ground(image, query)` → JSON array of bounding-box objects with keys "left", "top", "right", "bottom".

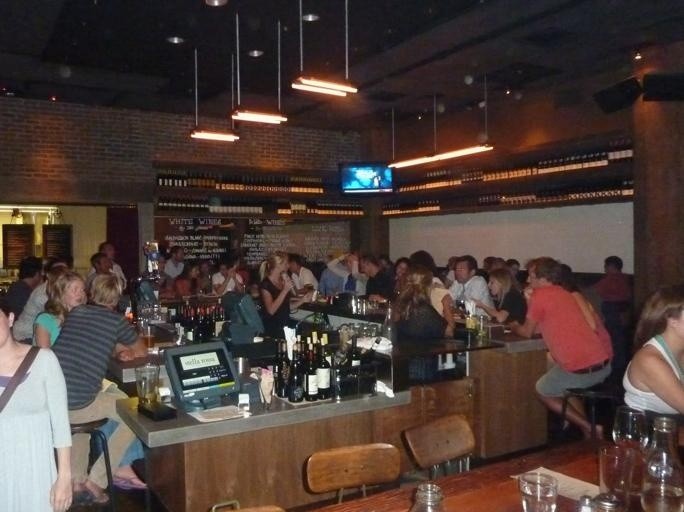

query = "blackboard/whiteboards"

[
  {"left": 154, "top": 217, "right": 358, "bottom": 268},
  {"left": 42, "top": 224, "right": 73, "bottom": 265},
  {"left": 388, "top": 196, "right": 641, "bottom": 278},
  {"left": 2, "top": 223, "right": 33, "bottom": 269}
]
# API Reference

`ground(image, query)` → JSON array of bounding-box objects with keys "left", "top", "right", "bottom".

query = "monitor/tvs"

[
  {"left": 222, "top": 292, "right": 264, "bottom": 331},
  {"left": 165, "top": 340, "right": 240, "bottom": 409},
  {"left": 339, "top": 160, "right": 394, "bottom": 196}
]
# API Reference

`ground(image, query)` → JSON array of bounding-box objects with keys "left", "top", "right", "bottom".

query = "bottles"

[
  {"left": 281, "top": 271, "right": 298, "bottom": 297},
  {"left": 272, "top": 331, "right": 361, "bottom": 402},
  {"left": 410, "top": 482, "right": 445, "bottom": 512},
  {"left": 350, "top": 295, "right": 379, "bottom": 316},
  {"left": 579, "top": 492, "right": 618, "bottom": 512},
  {"left": 168, "top": 299, "right": 225, "bottom": 342},
  {"left": 383, "top": 300, "right": 396, "bottom": 344},
  {"left": 641, "top": 416, "right": 684, "bottom": 512}
]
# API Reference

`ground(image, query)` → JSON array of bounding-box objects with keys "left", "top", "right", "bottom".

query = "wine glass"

[{"left": 600, "top": 405, "right": 649, "bottom": 512}]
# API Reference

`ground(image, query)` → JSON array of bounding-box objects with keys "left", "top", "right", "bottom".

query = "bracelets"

[{"left": 352, "top": 261, "right": 358, "bottom": 264}]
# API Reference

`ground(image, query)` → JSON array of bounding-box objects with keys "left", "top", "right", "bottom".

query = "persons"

[
  {"left": 0, "top": 287, "right": 74, "bottom": 512},
  {"left": 508, "top": 256, "right": 611, "bottom": 447},
  {"left": 590, "top": 256, "right": 631, "bottom": 302},
  {"left": 622, "top": 285, "right": 684, "bottom": 463},
  {"left": 32, "top": 271, "right": 148, "bottom": 491},
  {"left": 51, "top": 272, "right": 148, "bottom": 505},
  {"left": 3, "top": 239, "right": 526, "bottom": 344},
  {"left": 547, "top": 265, "right": 614, "bottom": 430}
]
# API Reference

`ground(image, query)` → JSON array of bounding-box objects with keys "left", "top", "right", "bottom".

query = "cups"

[
  {"left": 518, "top": 471, "right": 559, "bottom": 512},
  {"left": 143, "top": 326, "right": 156, "bottom": 349},
  {"left": 477, "top": 314, "right": 488, "bottom": 335},
  {"left": 135, "top": 362, "right": 160, "bottom": 402},
  {"left": 350, "top": 321, "right": 380, "bottom": 338}
]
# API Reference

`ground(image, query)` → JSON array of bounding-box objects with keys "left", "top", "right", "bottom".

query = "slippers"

[{"left": 81, "top": 476, "right": 148, "bottom": 503}]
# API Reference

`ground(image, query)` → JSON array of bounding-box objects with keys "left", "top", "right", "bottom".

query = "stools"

[
  {"left": 69, "top": 417, "right": 116, "bottom": 511},
  {"left": 560, "top": 386, "right": 618, "bottom": 439}
]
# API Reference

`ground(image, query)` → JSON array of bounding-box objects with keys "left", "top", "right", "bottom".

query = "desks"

[{"left": 302, "top": 442, "right": 645, "bottom": 512}]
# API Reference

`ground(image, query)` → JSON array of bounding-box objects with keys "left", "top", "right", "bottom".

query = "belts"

[{"left": 573, "top": 359, "right": 610, "bottom": 374}]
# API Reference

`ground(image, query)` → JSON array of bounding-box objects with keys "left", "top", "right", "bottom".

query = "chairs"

[
  {"left": 210, "top": 499, "right": 286, "bottom": 512},
  {"left": 305, "top": 443, "right": 402, "bottom": 504},
  {"left": 402, "top": 413, "right": 476, "bottom": 480}
]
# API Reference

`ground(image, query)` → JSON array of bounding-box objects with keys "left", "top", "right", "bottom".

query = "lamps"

[
  {"left": 191, "top": 48, "right": 240, "bottom": 143},
  {"left": 388, "top": 72, "right": 492, "bottom": 169},
  {"left": 290, "top": 2, "right": 358, "bottom": 97},
  {"left": 231, "top": 11, "right": 288, "bottom": 125}
]
